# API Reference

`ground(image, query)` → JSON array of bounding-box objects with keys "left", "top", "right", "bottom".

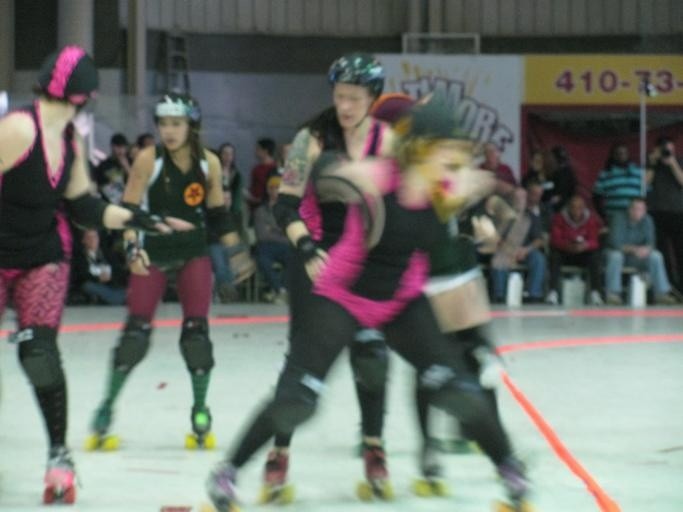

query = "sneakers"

[{"left": 543, "top": 287, "right": 678, "bottom": 306}]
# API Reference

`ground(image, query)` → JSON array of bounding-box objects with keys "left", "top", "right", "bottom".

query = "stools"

[
  {"left": 210, "top": 261, "right": 272, "bottom": 302},
  {"left": 471, "top": 258, "right": 648, "bottom": 306}
]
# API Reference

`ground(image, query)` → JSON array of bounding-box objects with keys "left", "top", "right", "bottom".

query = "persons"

[
  {"left": 204, "top": 92, "right": 531, "bottom": 512},
  {"left": 212, "top": 140, "right": 293, "bottom": 305},
  {"left": 457, "top": 138, "right": 683, "bottom": 308},
  {"left": 86, "top": 90, "right": 232, "bottom": 447},
  {"left": 404, "top": 316, "right": 498, "bottom": 495},
  {"left": 261, "top": 52, "right": 402, "bottom": 504},
  {"left": 0, "top": 44, "right": 197, "bottom": 505},
  {"left": 371, "top": 94, "right": 510, "bottom": 479}
]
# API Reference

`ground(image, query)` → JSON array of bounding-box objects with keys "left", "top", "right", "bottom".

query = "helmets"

[
  {"left": 327, "top": 52, "right": 389, "bottom": 99},
  {"left": 405, "top": 91, "right": 476, "bottom": 143},
  {"left": 37, "top": 44, "right": 101, "bottom": 104},
  {"left": 152, "top": 92, "right": 203, "bottom": 127}
]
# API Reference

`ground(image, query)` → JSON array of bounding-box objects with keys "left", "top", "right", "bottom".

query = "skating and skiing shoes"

[
  {"left": 353, "top": 437, "right": 396, "bottom": 502},
  {"left": 258, "top": 448, "right": 294, "bottom": 506},
  {"left": 198, "top": 457, "right": 242, "bottom": 512},
  {"left": 412, "top": 444, "right": 449, "bottom": 497},
  {"left": 42, "top": 444, "right": 77, "bottom": 506},
  {"left": 491, "top": 454, "right": 538, "bottom": 512},
  {"left": 184, "top": 403, "right": 218, "bottom": 451},
  {"left": 81, "top": 400, "right": 122, "bottom": 453}
]
